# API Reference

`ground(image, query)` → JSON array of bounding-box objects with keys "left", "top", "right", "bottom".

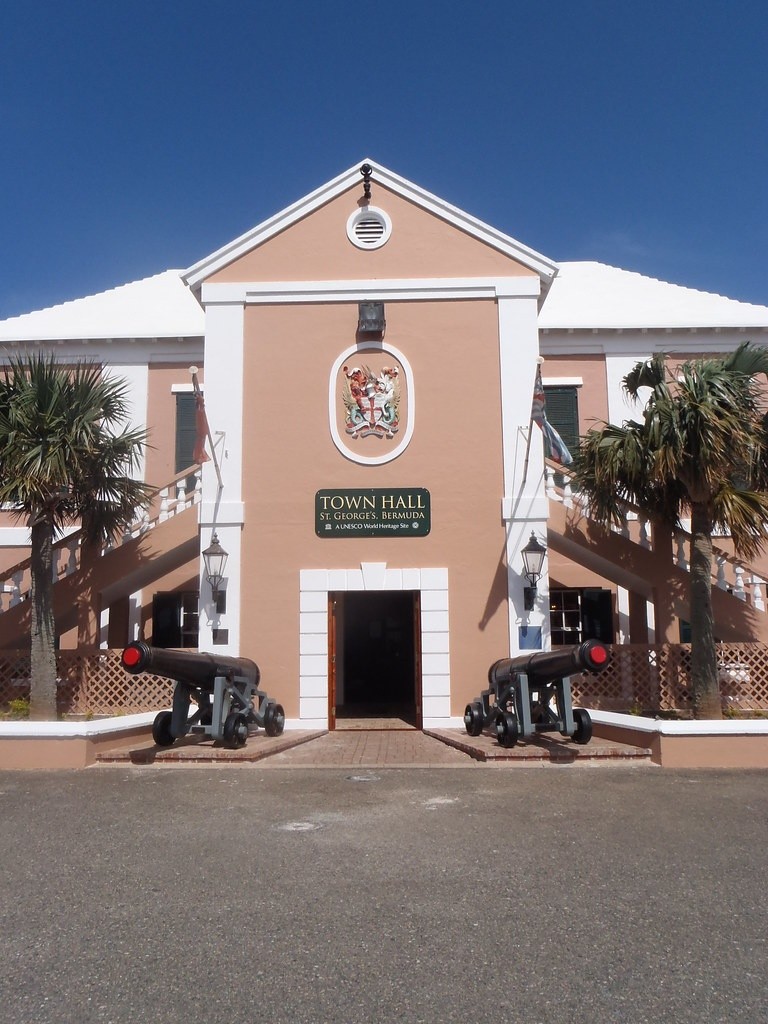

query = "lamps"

[
  {"left": 357, "top": 301, "right": 386, "bottom": 332},
  {"left": 520, "top": 529, "right": 546, "bottom": 611},
  {"left": 202, "top": 532, "right": 228, "bottom": 613}
]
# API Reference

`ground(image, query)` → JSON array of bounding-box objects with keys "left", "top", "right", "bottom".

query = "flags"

[
  {"left": 188, "top": 366, "right": 213, "bottom": 465},
  {"left": 531, "top": 355, "right": 574, "bottom": 465}
]
